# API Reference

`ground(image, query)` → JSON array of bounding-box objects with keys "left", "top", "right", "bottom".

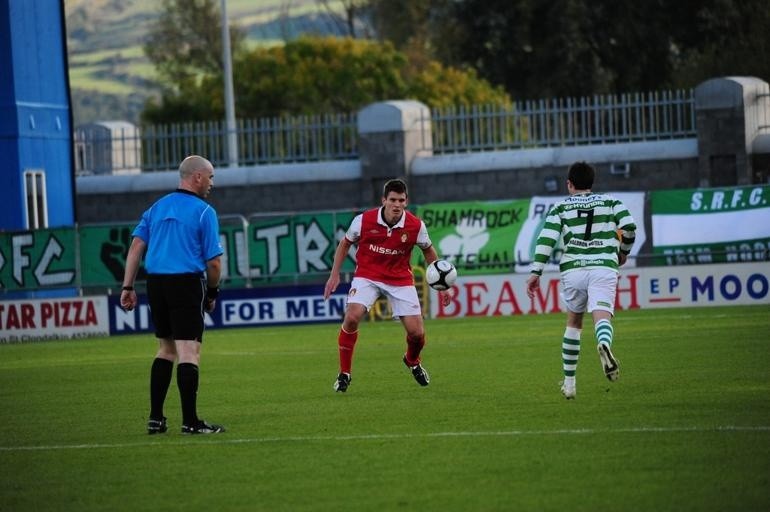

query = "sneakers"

[
  {"left": 334, "top": 369, "right": 351, "bottom": 392},
  {"left": 148, "top": 417, "right": 167, "bottom": 434},
  {"left": 181, "top": 419, "right": 225, "bottom": 434},
  {"left": 403, "top": 352, "right": 430, "bottom": 386},
  {"left": 560, "top": 384, "right": 575, "bottom": 399},
  {"left": 597, "top": 341, "right": 619, "bottom": 381}
]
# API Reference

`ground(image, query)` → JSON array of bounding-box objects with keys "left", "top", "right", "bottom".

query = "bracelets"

[
  {"left": 122, "top": 287, "right": 134, "bottom": 291},
  {"left": 206, "top": 286, "right": 218, "bottom": 298}
]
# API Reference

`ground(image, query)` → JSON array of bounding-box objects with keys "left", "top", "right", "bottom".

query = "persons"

[
  {"left": 120, "top": 155, "right": 227, "bottom": 434},
  {"left": 324, "top": 178, "right": 438, "bottom": 393},
  {"left": 526, "top": 160, "right": 637, "bottom": 399}
]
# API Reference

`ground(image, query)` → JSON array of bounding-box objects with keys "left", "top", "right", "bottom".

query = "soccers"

[{"left": 426, "top": 260, "right": 457, "bottom": 290}]
